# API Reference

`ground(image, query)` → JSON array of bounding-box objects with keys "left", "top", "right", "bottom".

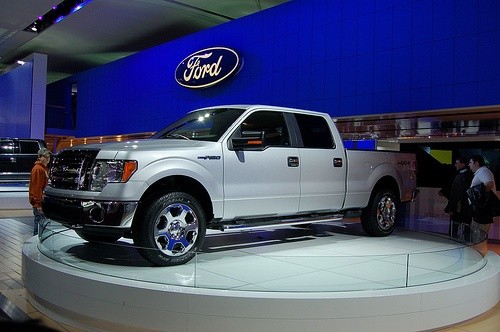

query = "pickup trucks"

[{"left": 43, "top": 106, "right": 416, "bottom": 267}]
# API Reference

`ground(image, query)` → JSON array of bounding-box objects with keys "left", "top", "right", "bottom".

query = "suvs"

[{"left": 0, "top": 137, "right": 48, "bottom": 181}]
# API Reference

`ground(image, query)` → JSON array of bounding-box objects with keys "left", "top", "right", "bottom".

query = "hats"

[{"left": 38, "top": 148, "right": 52, "bottom": 155}]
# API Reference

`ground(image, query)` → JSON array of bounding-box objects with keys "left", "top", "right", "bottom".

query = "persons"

[
  {"left": 448, "top": 157, "right": 473, "bottom": 241},
  {"left": 29, "top": 148, "right": 50, "bottom": 236},
  {"left": 466, "top": 154, "right": 497, "bottom": 234}
]
